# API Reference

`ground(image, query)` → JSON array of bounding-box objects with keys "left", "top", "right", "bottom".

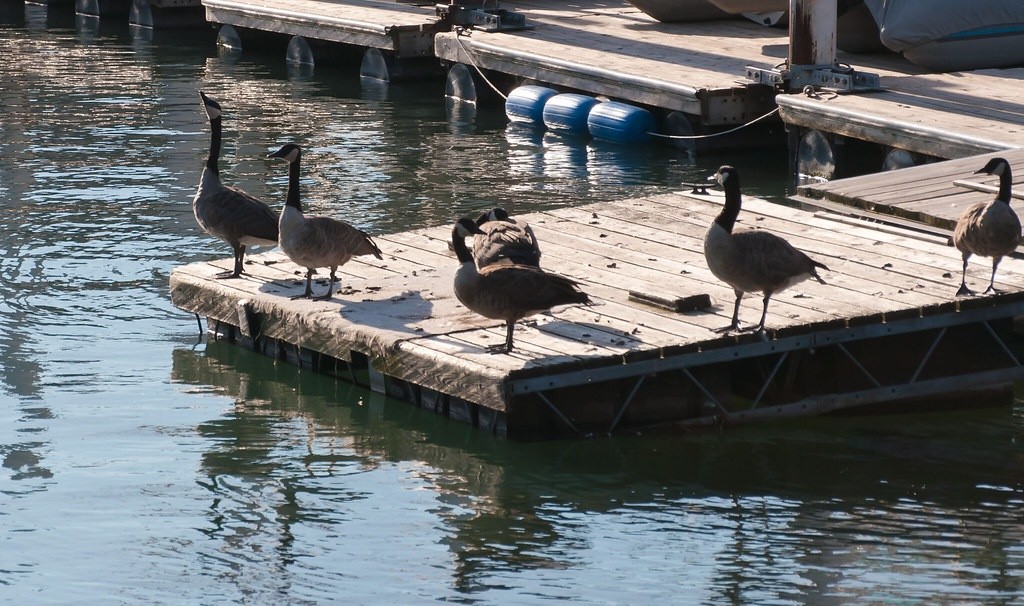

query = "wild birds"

[
  {"left": 703, "top": 165, "right": 831, "bottom": 336},
  {"left": 952, "top": 157, "right": 1022, "bottom": 299},
  {"left": 191, "top": 91, "right": 279, "bottom": 281},
  {"left": 451, "top": 207, "right": 597, "bottom": 356},
  {"left": 265, "top": 142, "right": 384, "bottom": 303}
]
